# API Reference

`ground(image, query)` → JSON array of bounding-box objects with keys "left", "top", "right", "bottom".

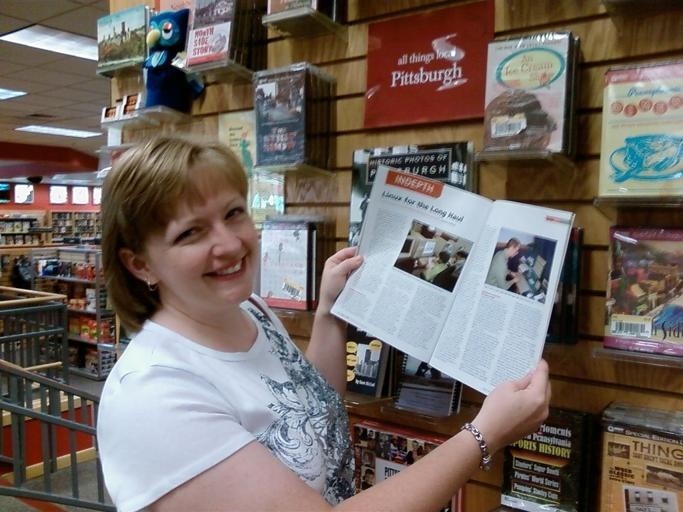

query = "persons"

[{"left": 96, "top": 136, "right": 551, "bottom": 512}]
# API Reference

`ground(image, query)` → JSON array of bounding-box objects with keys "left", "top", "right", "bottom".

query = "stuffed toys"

[{"left": 142, "top": 9, "right": 206, "bottom": 114}]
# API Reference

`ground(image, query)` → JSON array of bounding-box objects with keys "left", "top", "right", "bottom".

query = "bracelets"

[{"left": 460, "top": 419, "right": 495, "bottom": 471}]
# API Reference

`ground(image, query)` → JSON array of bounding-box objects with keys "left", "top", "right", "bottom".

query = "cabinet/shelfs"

[
  {"left": 47, "top": 210, "right": 103, "bottom": 244},
  {"left": 30, "top": 246, "right": 120, "bottom": 382},
  {"left": 0, "top": 209, "right": 51, "bottom": 287}
]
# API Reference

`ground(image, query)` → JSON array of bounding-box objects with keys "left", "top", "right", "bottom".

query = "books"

[
  {"left": 601, "top": 399, "right": 682, "bottom": 512},
  {"left": 481, "top": 28, "right": 584, "bottom": 160},
  {"left": 106, "top": 93, "right": 139, "bottom": 117},
  {"left": 286, "top": 1, "right": 349, "bottom": 26},
  {"left": 253, "top": 62, "right": 337, "bottom": 166},
  {"left": 187, "top": 1, "right": 267, "bottom": 71},
  {"left": 492, "top": 404, "right": 601, "bottom": 511},
  {"left": 602, "top": 224, "right": 683, "bottom": 356},
  {"left": 330, "top": 138, "right": 586, "bottom": 417},
  {"left": 596, "top": 62, "right": 682, "bottom": 205},
  {"left": 345, "top": 419, "right": 465, "bottom": 512},
  {"left": 260, "top": 216, "right": 337, "bottom": 311},
  {"left": 96, "top": 5, "right": 157, "bottom": 67},
  {"left": 219, "top": 111, "right": 285, "bottom": 218}
]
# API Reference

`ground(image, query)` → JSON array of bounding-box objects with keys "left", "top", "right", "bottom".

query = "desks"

[{"left": 344, "top": 392, "right": 509, "bottom": 511}]
